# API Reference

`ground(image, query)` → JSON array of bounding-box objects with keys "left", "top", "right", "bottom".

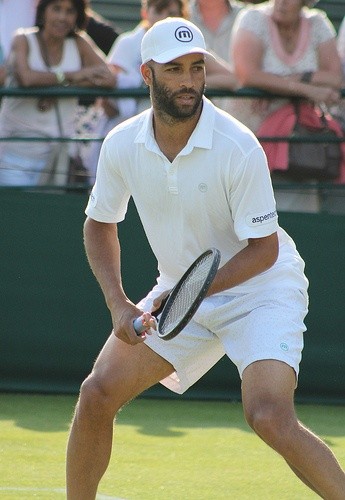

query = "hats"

[{"left": 139, "top": 17, "right": 216, "bottom": 65}]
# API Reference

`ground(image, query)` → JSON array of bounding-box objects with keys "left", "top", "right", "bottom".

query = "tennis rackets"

[{"left": 133, "top": 248, "right": 221, "bottom": 340}]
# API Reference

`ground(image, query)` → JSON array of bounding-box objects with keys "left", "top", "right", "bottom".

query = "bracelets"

[
  {"left": 300, "top": 72, "right": 312, "bottom": 84},
  {"left": 52, "top": 70, "right": 65, "bottom": 84}
]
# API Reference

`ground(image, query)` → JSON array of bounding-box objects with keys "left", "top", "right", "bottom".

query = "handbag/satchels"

[
  {"left": 275, "top": 91, "right": 342, "bottom": 180},
  {"left": 35, "top": 138, "right": 91, "bottom": 192}
]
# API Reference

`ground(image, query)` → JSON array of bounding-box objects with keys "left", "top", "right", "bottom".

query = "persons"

[
  {"left": 67, "top": 19, "right": 344, "bottom": 500},
  {"left": 1, "top": 1, "right": 345, "bottom": 183}
]
